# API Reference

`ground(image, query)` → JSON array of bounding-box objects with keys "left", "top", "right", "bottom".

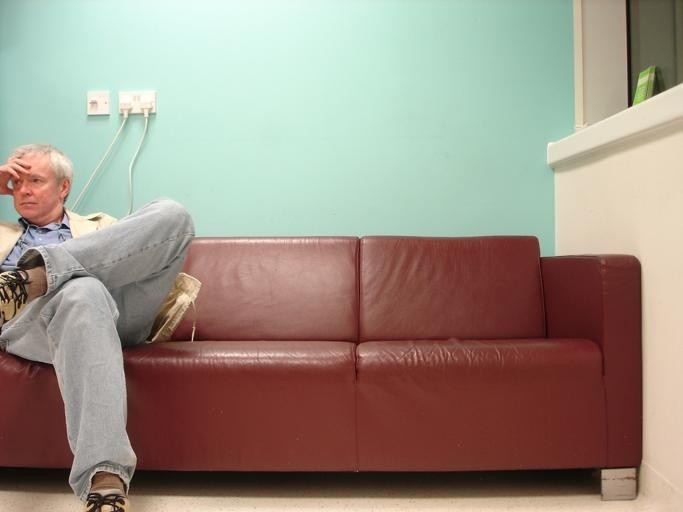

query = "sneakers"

[
  {"left": 0, "top": 272, "right": 25, "bottom": 324},
  {"left": 87, "top": 489, "right": 132, "bottom": 512}
]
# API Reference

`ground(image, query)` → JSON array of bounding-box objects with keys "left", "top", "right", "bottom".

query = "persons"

[{"left": 0, "top": 145, "right": 194, "bottom": 512}]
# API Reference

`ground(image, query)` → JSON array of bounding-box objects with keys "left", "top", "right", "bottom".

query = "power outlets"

[{"left": 118, "top": 91, "right": 156, "bottom": 115}]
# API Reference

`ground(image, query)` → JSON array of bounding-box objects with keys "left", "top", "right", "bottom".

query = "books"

[{"left": 633, "top": 66, "right": 656, "bottom": 105}]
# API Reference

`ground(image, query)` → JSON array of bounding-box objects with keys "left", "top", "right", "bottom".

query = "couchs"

[{"left": 0, "top": 236, "right": 643, "bottom": 501}]
